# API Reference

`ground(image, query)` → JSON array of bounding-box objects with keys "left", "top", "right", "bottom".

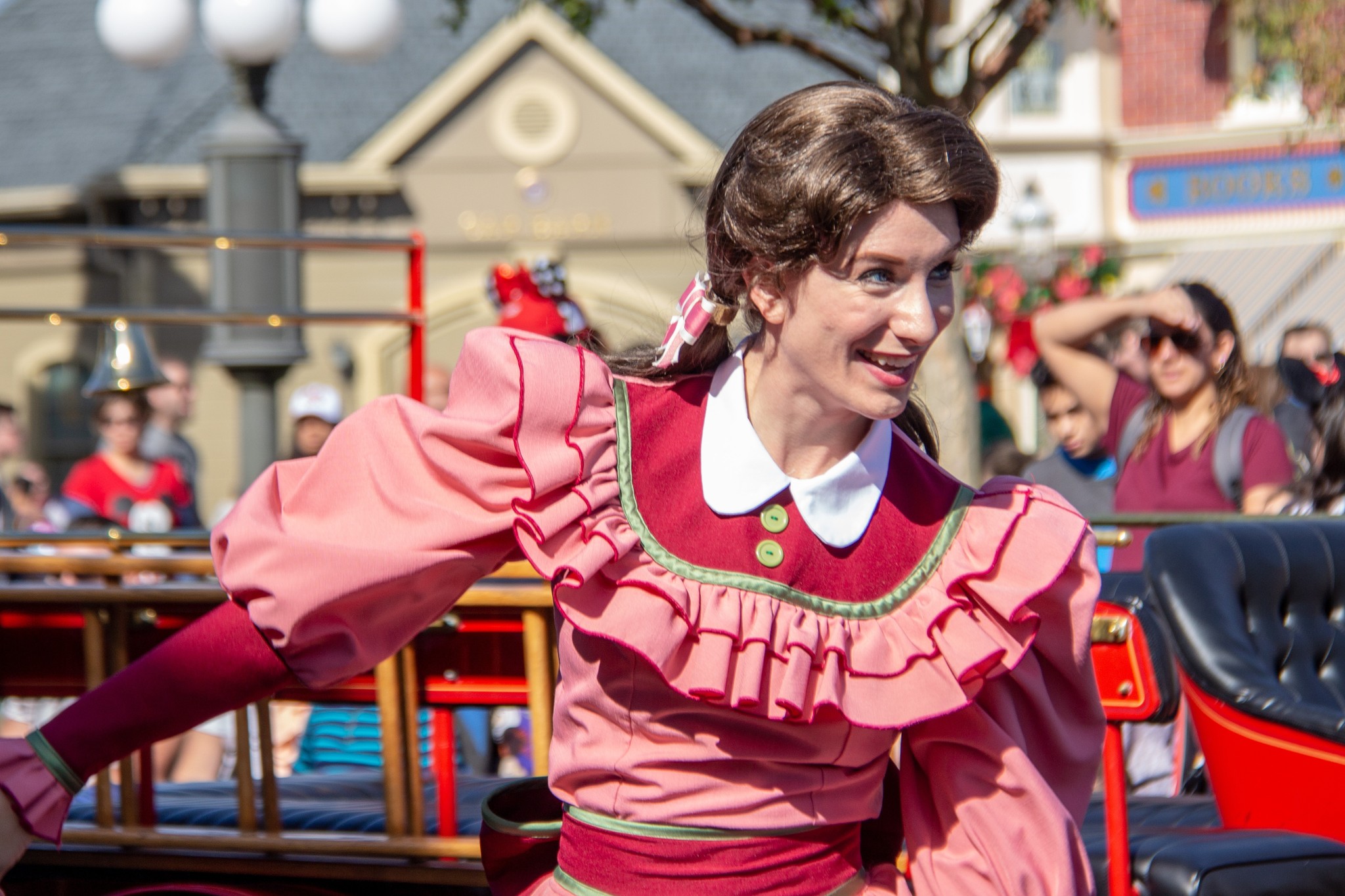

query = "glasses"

[{"left": 1137, "top": 331, "right": 1213, "bottom": 357}]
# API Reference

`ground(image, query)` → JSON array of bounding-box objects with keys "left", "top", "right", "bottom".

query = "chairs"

[{"left": 1143, "top": 520, "right": 1345, "bottom": 844}]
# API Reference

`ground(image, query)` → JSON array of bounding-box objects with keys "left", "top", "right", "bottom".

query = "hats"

[{"left": 292, "top": 383, "right": 343, "bottom": 423}]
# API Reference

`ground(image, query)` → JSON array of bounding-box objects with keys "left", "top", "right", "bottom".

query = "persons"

[
  {"left": 1020, "top": 359, "right": 1118, "bottom": 573},
  {"left": 61, "top": 388, "right": 194, "bottom": 582},
  {"left": 1032, "top": 282, "right": 1294, "bottom": 799},
  {"left": 1274, "top": 326, "right": 1345, "bottom": 459},
  {"left": 0, "top": 81, "right": 1107, "bottom": 896},
  {"left": 0, "top": 404, "right": 68, "bottom": 584},
  {"left": 137, "top": 359, "right": 196, "bottom": 485},
  {"left": 0, "top": 515, "right": 185, "bottom": 783},
  {"left": 288, "top": 383, "right": 341, "bottom": 458},
  {"left": 1262, "top": 391, "right": 1345, "bottom": 518}
]
